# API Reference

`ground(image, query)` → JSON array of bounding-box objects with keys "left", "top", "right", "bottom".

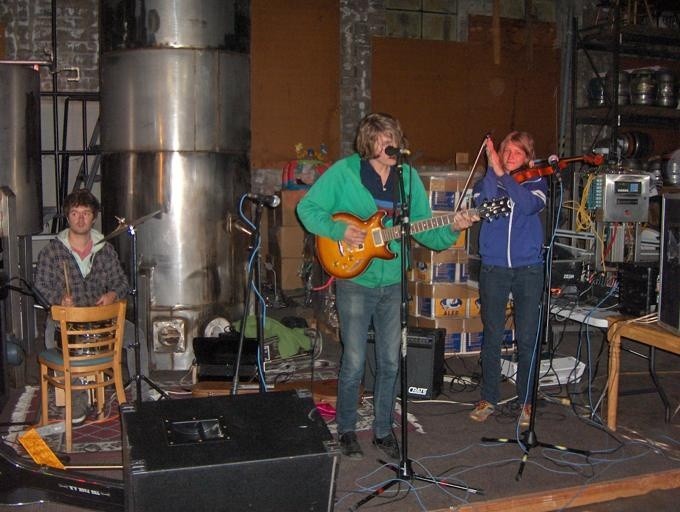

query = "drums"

[{"left": 53, "top": 323, "right": 115, "bottom": 359}]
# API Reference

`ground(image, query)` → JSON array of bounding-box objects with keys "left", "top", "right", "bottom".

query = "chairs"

[{"left": 36, "top": 298, "right": 129, "bottom": 455}]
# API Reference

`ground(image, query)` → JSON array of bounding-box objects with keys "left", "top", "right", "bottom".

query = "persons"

[
  {"left": 461, "top": 130, "right": 552, "bottom": 426},
  {"left": 34, "top": 187, "right": 130, "bottom": 424},
  {"left": 296, "top": 112, "right": 480, "bottom": 459}
]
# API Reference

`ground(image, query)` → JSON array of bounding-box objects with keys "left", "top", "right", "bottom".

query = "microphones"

[
  {"left": 385, "top": 146, "right": 411, "bottom": 155},
  {"left": 20, "top": 277, "right": 51, "bottom": 311},
  {"left": 548, "top": 155, "right": 562, "bottom": 182},
  {"left": 247, "top": 193, "right": 281, "bottom": 208}
]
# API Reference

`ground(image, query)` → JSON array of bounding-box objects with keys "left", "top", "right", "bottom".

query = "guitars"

[{"left": 315, "top": 197, "right": 513, "bottom": 279}]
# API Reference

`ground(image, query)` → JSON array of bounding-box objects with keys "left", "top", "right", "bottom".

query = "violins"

[{"left": 509, "top": 153, "right": 602, "bottom": 183}]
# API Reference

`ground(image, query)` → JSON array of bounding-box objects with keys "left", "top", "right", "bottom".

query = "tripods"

[
  {"left": 104, "top": 234, "right": 174, "bottom": 408},
  {"left": 482, "top": 173, "right": 592, "bottom": 482},
  {"left": 348, "top": 156, "right": 486, "bottom": 512}
]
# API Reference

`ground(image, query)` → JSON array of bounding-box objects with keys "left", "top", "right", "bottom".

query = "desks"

[{"left": 462, "top": 278, "right": 680, "bottom": 432}]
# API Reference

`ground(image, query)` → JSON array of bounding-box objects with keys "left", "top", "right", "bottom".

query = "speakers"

[
  {"left": 362, "top": 325, "right": 446, "bottom": 401},
  {"left": 658, "top": 193, "right": 680, "bottom": 337},
  {"left": 119, "top": 387, "right": 342, "bottom": 512}
]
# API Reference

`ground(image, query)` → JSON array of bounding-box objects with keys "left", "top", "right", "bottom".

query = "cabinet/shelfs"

[{"left": 567, "top": 3, "right": 680, "bottom": 203}]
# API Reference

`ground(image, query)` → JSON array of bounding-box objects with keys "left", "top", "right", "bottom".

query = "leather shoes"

[
  {"left": 339, "top": 430, "right": 365, "bottom": 459},
  {"left": 372, "top": 433, "right": 401, "bottom": 460}
]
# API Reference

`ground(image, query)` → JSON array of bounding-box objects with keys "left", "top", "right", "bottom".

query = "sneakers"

[
  {"left": 469, "top": 399, "right": 496, "bottom": 423},
  {"left": 71, "top": 392, "right": 89, "bottom": 424},
  {"left": 517, "top": 403, "right": 533, "bottom": 427}
]
